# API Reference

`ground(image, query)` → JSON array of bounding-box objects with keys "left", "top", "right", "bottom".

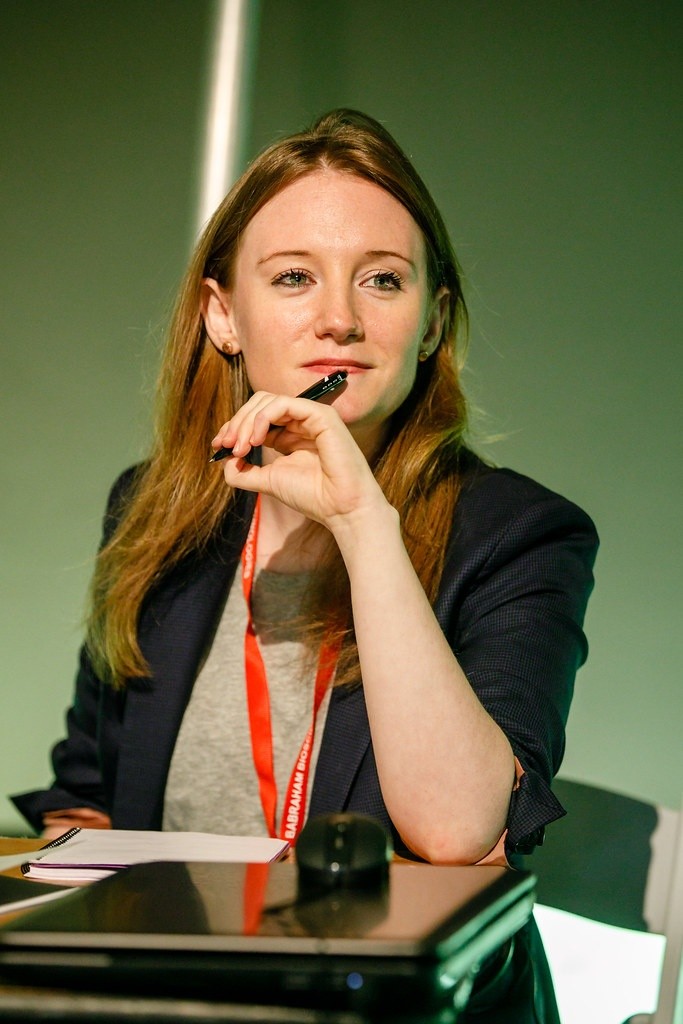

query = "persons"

[{"left": 7, "top": 108, "right": 601, "bottom": 1024}]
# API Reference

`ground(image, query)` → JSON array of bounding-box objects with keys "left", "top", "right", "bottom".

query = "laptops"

[{"left": 0, "top": 851, "right": 536, "bottom": 1011}]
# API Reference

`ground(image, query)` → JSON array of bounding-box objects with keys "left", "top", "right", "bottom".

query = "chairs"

[{"left": 526, "top": 779, "right": 682, "bottom": 1024}]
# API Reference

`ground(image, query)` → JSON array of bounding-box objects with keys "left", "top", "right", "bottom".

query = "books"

[{"left": 19, "top": 825, "right": 293, "bottom": 885}]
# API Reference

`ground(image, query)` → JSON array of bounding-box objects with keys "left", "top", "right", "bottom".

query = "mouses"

[{"left": 294, "top": 813, "right": 389, "bottom": 892}]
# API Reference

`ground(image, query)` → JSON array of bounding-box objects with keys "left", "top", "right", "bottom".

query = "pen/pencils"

[{"left": 208, "top": 369, "right": 350, "bottom": 464}]
